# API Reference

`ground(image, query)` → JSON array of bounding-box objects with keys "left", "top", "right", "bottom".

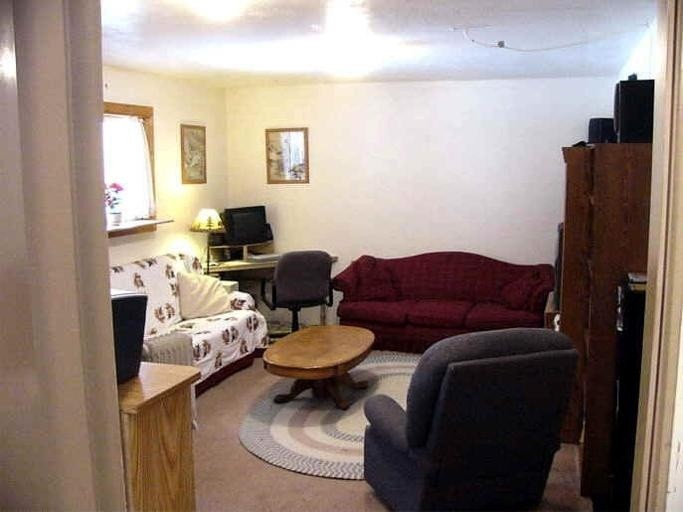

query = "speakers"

[{"left": 614, "top": 82, "right": 654, "bottom": 144}]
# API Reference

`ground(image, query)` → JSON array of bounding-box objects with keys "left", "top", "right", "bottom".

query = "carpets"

[{"left": 237, "top": 348, "right": 421, "bottom": 480}]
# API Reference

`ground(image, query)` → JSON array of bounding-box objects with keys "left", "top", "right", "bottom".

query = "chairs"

[
  {"left": 259, "top": 250, "right": 334, "bottom": 340},
  {"left": 363, "top": 327, "right": 578, "bottom": 511}
]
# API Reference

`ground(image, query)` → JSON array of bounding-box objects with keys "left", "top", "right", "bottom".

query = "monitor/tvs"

[{"left": 224, "top": 206, "right": 267, "bottom": 244}]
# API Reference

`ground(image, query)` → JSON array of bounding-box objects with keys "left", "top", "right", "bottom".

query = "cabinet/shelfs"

[{"left": 556, "top": 142, "right": 652, "bottom": 511}]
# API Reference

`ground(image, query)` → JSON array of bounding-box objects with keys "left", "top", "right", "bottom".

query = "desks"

[
  {"left": 202, "top": 254, "right": 338, "bottom": 326},
  {"left": 116, "top": 362, "right": 202, "bottom": 512}
]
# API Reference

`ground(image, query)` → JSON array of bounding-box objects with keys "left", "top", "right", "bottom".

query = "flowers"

[{"left": 104, "top": 183, "right": 124, "bottom": 209}]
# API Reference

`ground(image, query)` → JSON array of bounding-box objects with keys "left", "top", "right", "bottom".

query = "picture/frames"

[
  {"left": 264, "top": 127, "right": 309, "bottom": 185},
  {"left": 181, "top": 123, "right": 207, "bottom": 184}
]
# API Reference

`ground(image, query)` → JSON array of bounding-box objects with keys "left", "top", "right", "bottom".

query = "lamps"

[{"left": 189, "top": 208, "right": 224, "bottom": 277}]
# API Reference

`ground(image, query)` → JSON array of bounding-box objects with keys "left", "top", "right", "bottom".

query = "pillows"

[{"left": 176, "top": 271, "right": 232, "bottom": 321}]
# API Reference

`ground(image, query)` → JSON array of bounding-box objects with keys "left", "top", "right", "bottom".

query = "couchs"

[
  {"left": 333, "top": 251, "right": 556, "bottom": 353},
  {"left": 109, "top": 252, "right": 269, "bottom": 398}
]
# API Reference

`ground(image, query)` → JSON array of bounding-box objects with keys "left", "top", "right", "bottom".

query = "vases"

[{"left": 108, "top": 210, "right": 122, "bottom": 226}]
